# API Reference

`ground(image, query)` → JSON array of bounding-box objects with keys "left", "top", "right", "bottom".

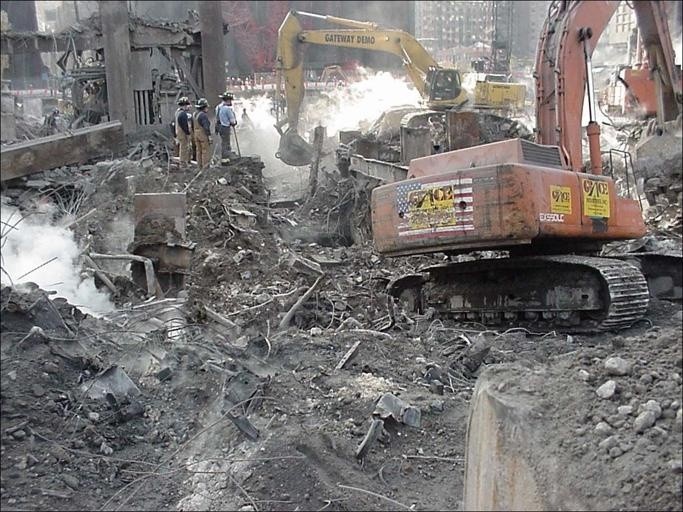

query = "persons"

[
  {"left": 174, "top": 96, "right": 192, "bottom": 170},
  {"left": 191, "top": 98, "right": 213, "bottom": 169},
  {"left": 214, "top": 90, "right": 237, "bottom": 164}
]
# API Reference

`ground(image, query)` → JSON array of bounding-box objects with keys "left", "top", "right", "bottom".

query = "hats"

[
  {"left": 176, "top": 96, "right": 190, "bottom": 106},
  {"left": 218, "top": 91, "right": 234, "bottom": 99},
  {"left": 194, "top": 98, "right": 209, "bottom": 110}
]
